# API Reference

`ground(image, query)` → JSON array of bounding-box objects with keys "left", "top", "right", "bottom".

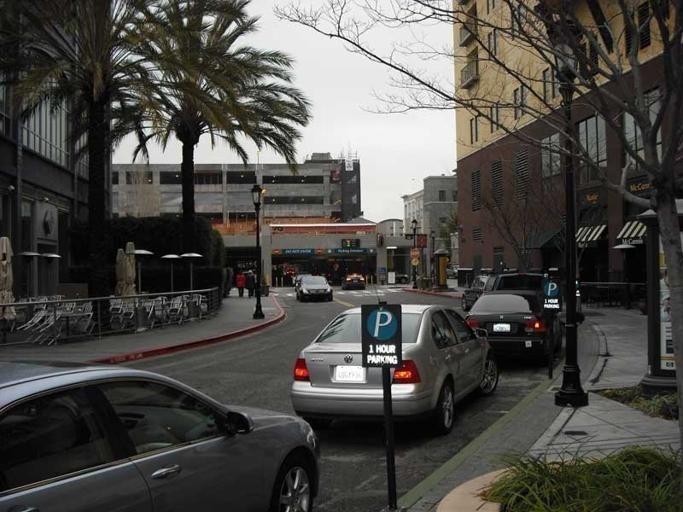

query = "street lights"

[
  {"left": 252, "top": 185, "right": 265, "bottom": 319},
  {"left": 411, "top": 219, "right": 418, "bottom": 289},
  {"left": 429, "top": 229, "right": 437, "bottom": 255},
  {"left": 546, "top": 20, "right": 587, "bottom": 407}
]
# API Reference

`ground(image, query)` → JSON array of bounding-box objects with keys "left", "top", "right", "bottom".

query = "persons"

[
  {"left": 272, "top": 263, "right": 295, "bottom": 288},
  {"left": 315, "top": 262, "right": 349, "bottom": 286},
  {"left": 411, "top": 265, "right": 420, "bottom": 290},
  {"left": 223, "top": 260, "right": 258, "bottom": 299}
]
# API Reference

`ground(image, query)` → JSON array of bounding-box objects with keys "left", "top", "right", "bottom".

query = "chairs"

[{"left": 13, "top": 294, "right": 208, "bottom": 345}]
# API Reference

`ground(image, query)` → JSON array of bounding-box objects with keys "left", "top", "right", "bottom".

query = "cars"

[
  {"left": 291, "top": 304, "right": 499, "bottom": 428},
  {"left": 295, "top": 273, "right": 333, "bottom": 301},
  {"left": 0, "top": 366, "right": 321, "bottom": 512},
  {"left": 341, "top": 273, "right": 365, "bottom": 290},
  {"left": 461, "top": 273, "right": 562, "bottom": 348}
]
